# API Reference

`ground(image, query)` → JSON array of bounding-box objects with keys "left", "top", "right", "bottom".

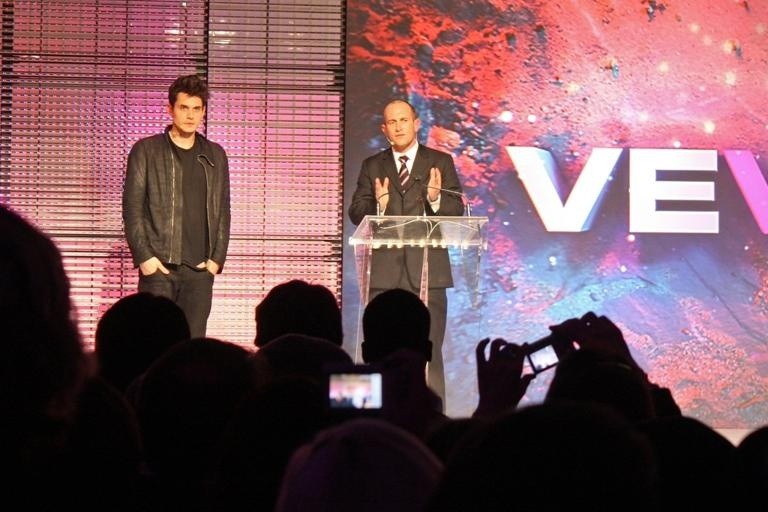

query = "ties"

[{"left": 397, "top": 156, "right": 410, "bottom": 191}]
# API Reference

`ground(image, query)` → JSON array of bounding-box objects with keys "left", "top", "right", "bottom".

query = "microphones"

[{"left": 373, "top": 176, "right": 472, "bottom": 216}]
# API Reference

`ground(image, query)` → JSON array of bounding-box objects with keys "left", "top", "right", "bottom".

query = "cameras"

[
  {"left": 323, "top": 371, "right": 384, "bottom": 412},
  {"left": 524, "top": 334, "right": 567, "bottom": 375}
]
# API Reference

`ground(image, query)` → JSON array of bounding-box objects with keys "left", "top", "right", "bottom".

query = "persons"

[
  {"left": 123, "top": 74, "right": 231, "bottom": 340},
  {"left": 42, "top": 280, "right": 768, "bottom": 512},
  {"left": 2, "top": 206, "right": 85, "bottom": 512},
  {"left": 348, "top": 100, "right": 464, "bottom": 414}
]
